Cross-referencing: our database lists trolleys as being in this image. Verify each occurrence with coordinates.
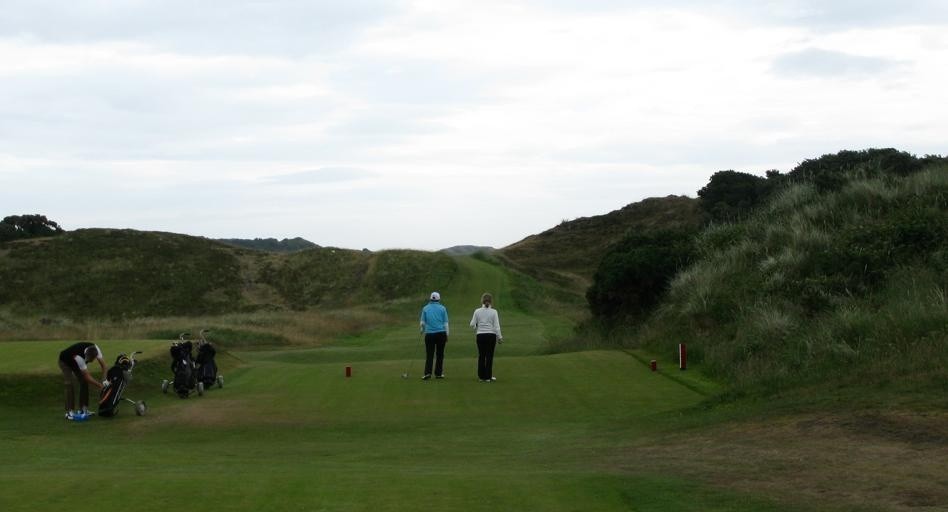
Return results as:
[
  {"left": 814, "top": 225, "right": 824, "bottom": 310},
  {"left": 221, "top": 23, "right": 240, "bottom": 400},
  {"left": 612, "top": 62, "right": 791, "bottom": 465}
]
[
  {"left": 160, "top": 332, "right": 204, "bottom": 400},
  {"left": 97, "top": 348, "right": 146, "bottom": 419},
  {"left": 193, "top": 326, "right": 224, "bottom": 390}
]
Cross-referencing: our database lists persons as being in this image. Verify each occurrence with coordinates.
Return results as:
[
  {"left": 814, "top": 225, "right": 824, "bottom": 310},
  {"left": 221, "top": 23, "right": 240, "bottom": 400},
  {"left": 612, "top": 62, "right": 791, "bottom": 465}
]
[
  {"left": 469, "top": 292, "right": 503, "bottom": 383},
  {"left": 419, "top": 291, "right": 449, "bottom": 380},
  {"left": 58, "top": 341, "right": 109, "bottom": 420}
]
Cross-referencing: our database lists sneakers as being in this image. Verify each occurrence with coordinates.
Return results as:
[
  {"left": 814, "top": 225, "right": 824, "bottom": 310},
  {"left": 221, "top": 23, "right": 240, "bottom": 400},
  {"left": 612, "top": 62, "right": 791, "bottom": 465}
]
[
  {"left": 477, "top": 377, "right": 496, "bottom": 382},
  {"left": 420, "top": 374, "right": 446, "bottom": 380},
  {"left": 64, "top": 408, "right": 96, "bottom": 420}
]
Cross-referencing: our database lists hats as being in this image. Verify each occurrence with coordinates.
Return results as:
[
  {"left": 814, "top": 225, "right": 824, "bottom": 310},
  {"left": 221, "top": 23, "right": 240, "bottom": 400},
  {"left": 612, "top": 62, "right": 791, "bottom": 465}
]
[{"left": 429, "top": 292, "right": 442, "bottom": 301}]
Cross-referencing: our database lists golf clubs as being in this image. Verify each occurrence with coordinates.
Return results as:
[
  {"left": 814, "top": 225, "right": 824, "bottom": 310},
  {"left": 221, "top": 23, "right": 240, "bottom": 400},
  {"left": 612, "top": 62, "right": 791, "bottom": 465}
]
[
  {"left": 401, "top": 334, "right": 421, "bottom": 378},
  {"left": 118, "top": 352, "right": 129, "bottom": 364}
]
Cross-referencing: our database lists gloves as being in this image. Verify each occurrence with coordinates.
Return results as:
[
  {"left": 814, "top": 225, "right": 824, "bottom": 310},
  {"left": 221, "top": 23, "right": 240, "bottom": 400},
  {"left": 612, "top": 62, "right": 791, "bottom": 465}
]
[{"left": 498, "top": 337, "right": 505, "bottom": 344}]
